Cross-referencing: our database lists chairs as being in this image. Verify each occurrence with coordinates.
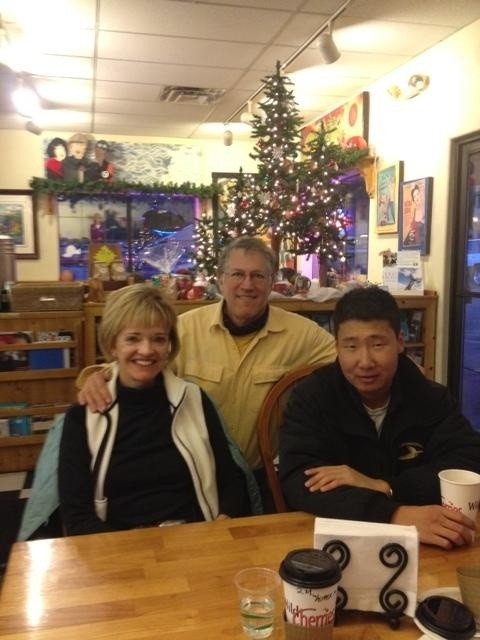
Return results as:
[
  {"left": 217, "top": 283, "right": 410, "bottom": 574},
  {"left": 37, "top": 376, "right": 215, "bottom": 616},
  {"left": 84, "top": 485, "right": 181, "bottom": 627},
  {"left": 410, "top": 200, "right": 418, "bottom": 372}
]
[{"left": 257, "top": 364, "right": 324, "bottom": 511}]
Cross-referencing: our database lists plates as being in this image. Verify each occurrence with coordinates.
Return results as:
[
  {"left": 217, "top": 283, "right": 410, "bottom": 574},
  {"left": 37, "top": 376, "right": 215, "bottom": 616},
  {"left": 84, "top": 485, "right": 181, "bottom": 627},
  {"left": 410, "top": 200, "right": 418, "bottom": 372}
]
[{"left": 415, "top": 587, "right": 480, "bottom": 639}]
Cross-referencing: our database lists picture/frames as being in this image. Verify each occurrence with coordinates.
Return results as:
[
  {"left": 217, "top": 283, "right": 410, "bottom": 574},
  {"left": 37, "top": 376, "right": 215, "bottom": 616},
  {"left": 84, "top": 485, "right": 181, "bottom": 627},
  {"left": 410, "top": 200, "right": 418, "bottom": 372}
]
[
  {"left": 374, "top": 161, "right": 403, "bottom": 235},
  {"left": 0, "top": 189, "right": 39, "bottom": 260},
  {"left": 397, "top": 177, "right": 433, "bottom": 256},
  {"left": 212, "top": 172, "right": 297, "bottom": 276}
]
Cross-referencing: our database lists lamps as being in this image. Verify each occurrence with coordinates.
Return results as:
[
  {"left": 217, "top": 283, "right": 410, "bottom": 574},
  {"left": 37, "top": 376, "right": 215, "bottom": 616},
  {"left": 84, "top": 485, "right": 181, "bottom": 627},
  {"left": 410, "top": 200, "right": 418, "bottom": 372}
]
[
  {"left": 223, "top": 131, "right": 232, "bottom": 144},
  {"left": 316, "top": 21, "right": 340, "bottom": 64},
  {"left": 26, "top": 122, "right": 42, "bottom": 135},
  {"left": 240, "top": 101, "right": 259, "bottom": 127}
]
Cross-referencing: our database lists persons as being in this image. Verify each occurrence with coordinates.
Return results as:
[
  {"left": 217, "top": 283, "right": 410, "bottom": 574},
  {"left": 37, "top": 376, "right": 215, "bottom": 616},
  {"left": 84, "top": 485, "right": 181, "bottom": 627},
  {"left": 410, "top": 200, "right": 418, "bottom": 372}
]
[
  {"left": 74, "top": 232, "right": 339, "bottom": 514},
  {"left": 277, "top": 287, "right": 480, "bottom": 551},
  {"left": 93, "top": 139, "right": 113, "bottom": 186},
  {"left": 404, "top": 184, "right": 425, "bottom": 245},
  {"left": 57, "top": 284, "right": 251, "bottom": 536},
  {"left": 46, "top": 137, "right": 66, "bottom": 180},
  {"left": 63, "top": 133, "right": 110, "bottom": 186}
]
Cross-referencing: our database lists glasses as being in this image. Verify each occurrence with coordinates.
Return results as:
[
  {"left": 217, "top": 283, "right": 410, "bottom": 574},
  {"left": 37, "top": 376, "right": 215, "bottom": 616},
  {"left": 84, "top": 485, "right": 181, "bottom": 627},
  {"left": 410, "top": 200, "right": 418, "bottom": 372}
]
[{"left": 222, "top": 269, "right": 275, "bottom": 284}]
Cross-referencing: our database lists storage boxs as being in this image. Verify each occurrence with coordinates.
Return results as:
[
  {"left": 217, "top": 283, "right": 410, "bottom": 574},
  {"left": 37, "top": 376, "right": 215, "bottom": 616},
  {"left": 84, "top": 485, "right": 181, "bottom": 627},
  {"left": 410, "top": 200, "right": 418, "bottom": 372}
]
[{"left": 10, "top": 281, "right": 83, "bottom": 311}]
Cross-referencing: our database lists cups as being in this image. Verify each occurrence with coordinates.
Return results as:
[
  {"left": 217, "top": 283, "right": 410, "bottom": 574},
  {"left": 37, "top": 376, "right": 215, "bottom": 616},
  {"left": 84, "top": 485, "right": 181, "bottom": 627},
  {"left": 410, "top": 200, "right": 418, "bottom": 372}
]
[
  {"left": 456, "top": 565, "right": 480, "bottom": 626},
  {"left": 232, "top": 566, "right": 281, "bottom": 637},
  {"left": 278, "top": 549, "right": 342, "bottom": 639},
  {"left": 437, "top": 469, "right": 480, "bottom": 526}
]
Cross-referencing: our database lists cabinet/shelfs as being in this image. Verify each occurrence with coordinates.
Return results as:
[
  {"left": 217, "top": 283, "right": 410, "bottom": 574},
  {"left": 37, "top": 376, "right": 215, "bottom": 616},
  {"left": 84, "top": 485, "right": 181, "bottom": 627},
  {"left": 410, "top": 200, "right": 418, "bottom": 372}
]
[
  {"left": 0, "top": 310, "right": 86, "bottom": 474},
  {"left": 87, "top": 290, "right": 438, "bottom": 381}
]
